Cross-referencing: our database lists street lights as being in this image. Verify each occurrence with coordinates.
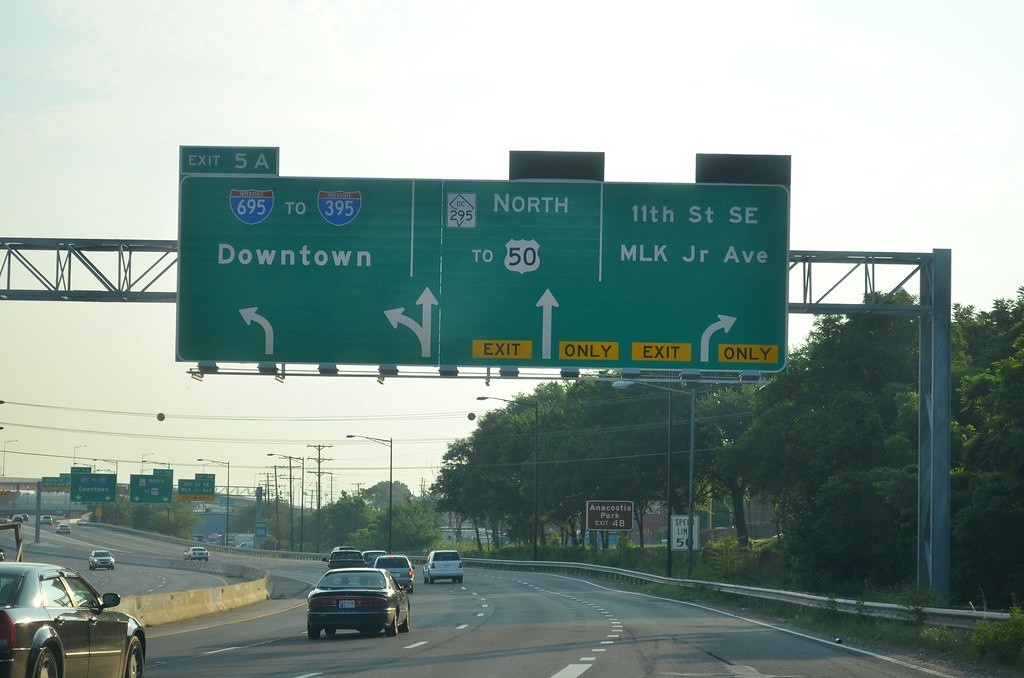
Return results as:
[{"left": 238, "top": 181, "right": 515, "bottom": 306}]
[
  {"left": 196, "top": 458, "right": 230, "bottom": 547},
  {"left": 345, "top": 434, "right": 393, "bottom": 554},
  {"left": 611, "top": 380, "right": 697, "bottom": 581},
  {"left": 2, "top": 439, "right": 19, "bottom": 478},
  {"left": 141, "top": 460, "right": 170, "bottom": 537},
  {"left": 92, "top": 458, "right": 118, "bottom": 476},
  {"left": 73, "top": 444, "right": 88, "bottom": 464},
  {"left": 475, "top": 396, "right": 539, "bottom": 562},
  {"left": 74, "top": 462, "right": 95, "bottom": 473},
  {"left": 267, "top": 453, "right": 304, "bottom": 553}
]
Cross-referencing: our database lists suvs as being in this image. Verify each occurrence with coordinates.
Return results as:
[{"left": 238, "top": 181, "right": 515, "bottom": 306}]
[
  {"left": 361, "top": 550, "right": 388, "bottom": 568},
  {"left": 423, "top": 549, "right": 463, "bottom": 584},
  {"left": 333, "top": 545, "right": 356, "bottom": 550},
  {"left": 89, "top": 549, "right": 115, "bottom": 571},
  {"left": 321, "top": 550, "right": 367, "bottom": 570},
  {"left": 374, "top": 555, "right": 415, "bottom": 593}
]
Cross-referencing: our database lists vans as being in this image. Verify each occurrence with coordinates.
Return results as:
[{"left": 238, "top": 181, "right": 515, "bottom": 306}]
[{"left": 236, "top": 542, "right": 253, "bottom": 548}]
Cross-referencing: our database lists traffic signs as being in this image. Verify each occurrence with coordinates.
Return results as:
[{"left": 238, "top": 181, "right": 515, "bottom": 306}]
[
  {"left": 443, "top": 180, "right": 601, "bottom": 367},
  {"left": 129, "top": 474, "right": 172, "bottom": 505},
  {"left": 70, "top": 473, "right": 117, "bottom": 503},
  {"left": 174, "top": 174, "right": 443, "bottom": 368},
  {"left": 600, "top": 180, "right": 790, "bottom": 373}
]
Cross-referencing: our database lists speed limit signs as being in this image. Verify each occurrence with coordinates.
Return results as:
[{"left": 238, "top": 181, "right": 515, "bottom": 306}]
[{"left": 670, "top": 514, "right": 701, "bottom": 552}]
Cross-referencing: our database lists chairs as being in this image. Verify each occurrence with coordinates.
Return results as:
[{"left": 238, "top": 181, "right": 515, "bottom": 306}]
[
  {"left": 399, "top": 560, "right": 406, "bottom": 568},
  {"left": 341, "top": 577, "right": 350, "bottom": 586},
  {"left": 359, "top": 576, "right": 369, "bottom": 586},
  {"left": 199, "top": 549, "right": 203, "bottom": 551},
  {"left": 451, "top": 555, "right": 458, "bottom": 560}
]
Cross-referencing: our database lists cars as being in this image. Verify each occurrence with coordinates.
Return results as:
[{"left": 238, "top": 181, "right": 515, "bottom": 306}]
[
  {"left": 307, "top": 567, "right": 411, "bottom": 639},
  {"left": 55, "top": 523, "right": 71, "bottom": 534},
  {"left": 39, "top": 515, "right": 53, "bottom": 526},
  {"left": 11, "top": 514, "right": 24, "bottom": 523},
  {"left": 183, "top": 547, "right": 209, "bottom": 562},
  {"left": 22, "top": 514, "right": 29, "bottom": 522},
  {"left": 0, "top": 561, "right": 147, "bottom": 678}
]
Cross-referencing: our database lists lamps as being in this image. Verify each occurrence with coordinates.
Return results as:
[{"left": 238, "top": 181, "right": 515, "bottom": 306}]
[
  {"left": 679, "top": 369, "right": 703, "bottom": 381},
  {"left": 378, "top": 364, "right": 399, "bottom": 375},
  {"left": 197, "top": 361, "right": 219, "bottom": 373},
  {"left": 257, "top": 362, "right": 279, "bottom": 374},
  {"left": 499, "top": 366, "right": 519, "bottom": 378},
  {"left": 317, "top": 364, "right": 339, "bottom": 375},
  {"left": 438, "top": 366, "right": 459, "bottom": 377},
  {"left": 560, "top": 368, "right": 580, "bottom": 377},
  {"left": 739, "top": 371, "right": 760, "bottom": 382},
  {"left": 621, "top": 367, "right": 641, "bottom": 378}
]
